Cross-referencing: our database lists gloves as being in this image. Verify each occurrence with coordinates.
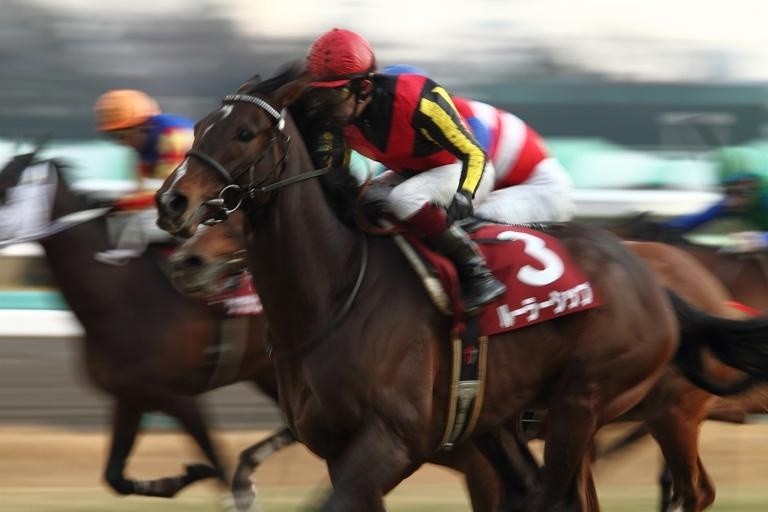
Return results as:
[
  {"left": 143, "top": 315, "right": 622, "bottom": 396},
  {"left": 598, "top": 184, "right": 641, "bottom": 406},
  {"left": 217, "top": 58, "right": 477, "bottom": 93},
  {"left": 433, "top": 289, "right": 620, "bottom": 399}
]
[{"left": 445, "top": 188, "right": 475, "bottom": 228}]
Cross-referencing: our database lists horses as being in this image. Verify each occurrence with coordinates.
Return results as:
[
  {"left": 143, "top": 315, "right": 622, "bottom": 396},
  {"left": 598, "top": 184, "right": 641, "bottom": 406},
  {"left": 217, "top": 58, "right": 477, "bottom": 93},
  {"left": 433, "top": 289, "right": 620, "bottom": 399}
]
[
  {"left": 0, "top": 148, "right": 278, "bottom": 500},
  {"left": 154, "top": 72, "right": 767, "bottom": 512},
  {"left": 548, "top": 214, "right": 767, "bottom": 512}
]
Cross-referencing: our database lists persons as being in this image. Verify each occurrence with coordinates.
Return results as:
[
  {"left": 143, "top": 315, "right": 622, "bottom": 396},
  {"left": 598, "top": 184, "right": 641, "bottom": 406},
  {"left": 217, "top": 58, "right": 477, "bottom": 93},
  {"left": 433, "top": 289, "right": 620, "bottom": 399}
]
[
  {"left": 302, "top": 26, "right": 575, "bottom": 326},
  {"left": 94, "top": 88, "right": 265, "bottom": 318},
  {"left": 640, "top": 141, "right": 766, "bottom": 256}
]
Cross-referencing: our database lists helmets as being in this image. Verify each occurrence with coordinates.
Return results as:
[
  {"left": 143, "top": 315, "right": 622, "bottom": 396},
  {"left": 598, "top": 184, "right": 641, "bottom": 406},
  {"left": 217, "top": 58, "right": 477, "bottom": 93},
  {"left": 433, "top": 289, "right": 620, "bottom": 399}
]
[
  {"left": 297, "top": 26, "right": 378, "bottom": 88},
  {"left": 92, "top": 89, "right": 161, "bottom": 133}
]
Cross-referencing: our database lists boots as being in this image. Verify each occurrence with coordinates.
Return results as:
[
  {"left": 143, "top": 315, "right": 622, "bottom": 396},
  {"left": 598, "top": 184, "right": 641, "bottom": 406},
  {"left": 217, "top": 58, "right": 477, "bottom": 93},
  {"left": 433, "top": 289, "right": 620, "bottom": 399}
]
[{"left": 428, "top": 221, "right": 508, "bottom": 314}]
